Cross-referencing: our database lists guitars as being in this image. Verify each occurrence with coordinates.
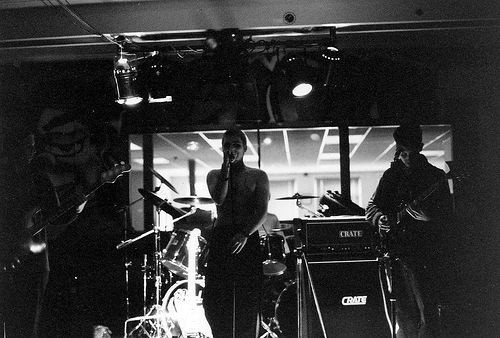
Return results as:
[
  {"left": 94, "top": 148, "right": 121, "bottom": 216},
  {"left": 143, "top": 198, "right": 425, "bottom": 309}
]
[
  {"left": 382, "top": 165, "right": 466, "bottom": 241},
  {"left": 0, "top": 160, "right": 132, "bottom": 271}
]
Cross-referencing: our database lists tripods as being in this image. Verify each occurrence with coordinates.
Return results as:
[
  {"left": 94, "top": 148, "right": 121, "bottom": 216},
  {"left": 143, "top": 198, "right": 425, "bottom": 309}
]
[{"left": 124, "top": 204, "right": 183, "bottom": 338}]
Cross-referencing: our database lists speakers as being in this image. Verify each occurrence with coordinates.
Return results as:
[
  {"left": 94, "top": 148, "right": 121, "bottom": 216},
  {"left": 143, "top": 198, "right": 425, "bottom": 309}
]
[{"left": 304, "top": 254, "right": 396, "bottom": 337}]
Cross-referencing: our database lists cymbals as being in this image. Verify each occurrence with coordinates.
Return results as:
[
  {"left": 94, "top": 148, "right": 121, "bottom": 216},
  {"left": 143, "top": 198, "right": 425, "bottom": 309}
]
[
  {"left": 274, "top": 194, "right": 321, "bottom": 201},
  {"left": 173, "top": 196, "right": 214, "bottom": 206},
  {"left": 144, "top": 163, "right": 179, "bottom": 194},
  {"left": 137, "top": 188, "right": 186, "bottom": 218}
]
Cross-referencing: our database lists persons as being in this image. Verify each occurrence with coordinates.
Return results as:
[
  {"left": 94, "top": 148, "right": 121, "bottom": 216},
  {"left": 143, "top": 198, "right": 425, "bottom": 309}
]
[
  {"left": 365, "top": 128, "right": 454, "bottom": 338},
  {"left": 206, "top": 126, "right": 290, "bottom": 338},
  {"left": 0, "top": 129, "right": 89, "bottom": 338}
]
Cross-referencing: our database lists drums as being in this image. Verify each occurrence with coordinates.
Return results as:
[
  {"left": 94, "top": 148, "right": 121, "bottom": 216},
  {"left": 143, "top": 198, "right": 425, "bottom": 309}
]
[
  {"left": 161, "top": 279, "right": 214, "bottom": 338},
  {"left": 259, "top": 232, "right": 288, "bottom": 277},
  {"left": 156, "top": 228, "right": 210, "bottom": 279}
]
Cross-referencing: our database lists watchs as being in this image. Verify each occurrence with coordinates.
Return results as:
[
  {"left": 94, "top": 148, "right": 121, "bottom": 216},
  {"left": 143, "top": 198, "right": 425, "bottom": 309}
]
[{"left": 219, "top": 173, "right": 230, "bottom": 182}]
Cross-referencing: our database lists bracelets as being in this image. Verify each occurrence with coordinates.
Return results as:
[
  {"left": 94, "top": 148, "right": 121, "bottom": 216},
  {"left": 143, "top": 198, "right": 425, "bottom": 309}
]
[{"left": 241, "top": 228, "right": 250, "bottom": 237}]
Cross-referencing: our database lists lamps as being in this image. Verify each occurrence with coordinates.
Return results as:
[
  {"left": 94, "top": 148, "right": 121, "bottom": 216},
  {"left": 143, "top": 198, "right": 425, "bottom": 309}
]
[
  {"left": 202, "top": 33, "right": 224, "bottom": 54},
  {"left": 113, "top": 50, "right": 144, "bottom": 106},
  {"left": 143, "top": 51, "right": 173, "bottom": 103},
  {"left": 319, "top": 28, "right": 346, "bottom": 63},
  {"left": 276, "top": 45, "right": 313, "bottom": 97}
]
